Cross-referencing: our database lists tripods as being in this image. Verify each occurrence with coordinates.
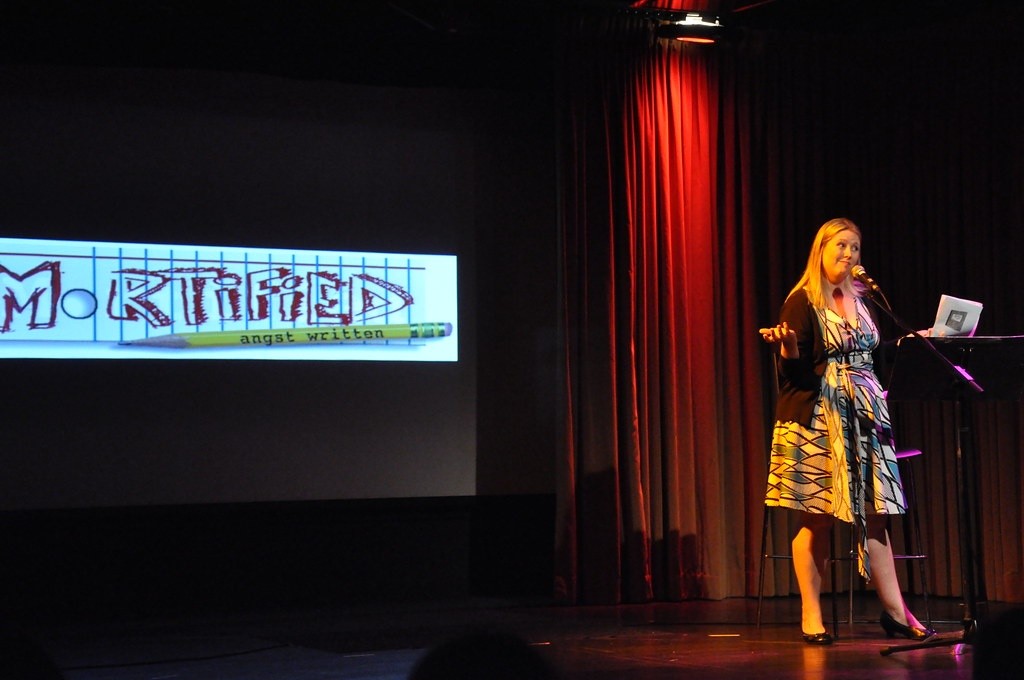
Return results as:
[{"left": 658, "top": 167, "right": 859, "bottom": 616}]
[{"left": 863, "top": 287, "right": 986, "bottom": 656}]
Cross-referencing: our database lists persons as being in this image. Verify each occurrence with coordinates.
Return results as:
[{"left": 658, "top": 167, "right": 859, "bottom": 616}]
[
  {"left": 408, "top": 632, "right": 557, "bottom": 680},
  {"left": 759, "top": 217, "right": 944, "bottom": 644},
  {"left": 0, "top": 612, "right": 62, "bottom": 680},
  {"left": 972, "top": 608, "right": 1024, "bottom": 679}
]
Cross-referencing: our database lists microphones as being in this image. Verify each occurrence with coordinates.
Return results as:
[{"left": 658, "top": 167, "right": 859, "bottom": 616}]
[{"left": 852, "top": 265, "right": 881, "bottom": 292}]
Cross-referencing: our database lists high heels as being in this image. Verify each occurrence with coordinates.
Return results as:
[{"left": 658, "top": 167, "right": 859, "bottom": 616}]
[
  {"left": 802, "top": 621, "right": 832, "bottom": 646},
  {"left": 880, "top": 610, "right": 938, "bottom": 640}
]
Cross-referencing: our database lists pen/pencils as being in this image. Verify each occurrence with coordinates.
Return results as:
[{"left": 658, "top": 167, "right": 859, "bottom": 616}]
[{"left": 116, "top": 320, "right": 455, "bottom": 350}]
